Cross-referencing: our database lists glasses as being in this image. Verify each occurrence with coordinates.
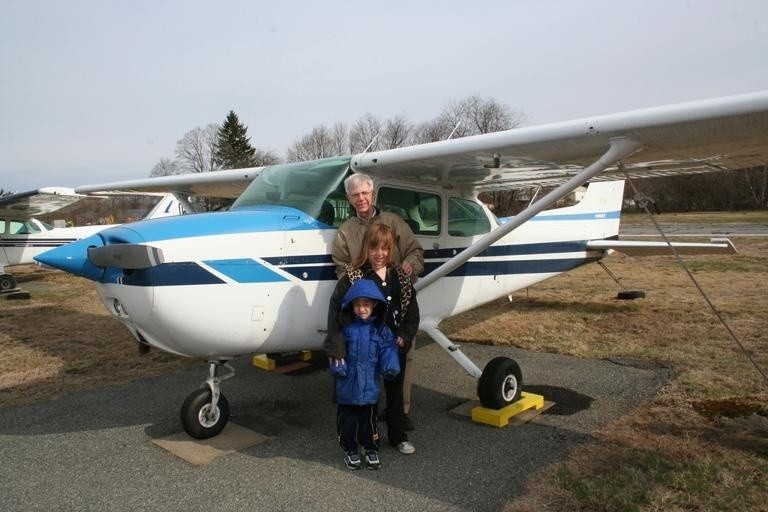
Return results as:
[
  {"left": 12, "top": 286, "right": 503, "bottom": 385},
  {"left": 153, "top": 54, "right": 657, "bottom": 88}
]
[{"left": 347, "top": 191, "right": 371, "bottom": 197}]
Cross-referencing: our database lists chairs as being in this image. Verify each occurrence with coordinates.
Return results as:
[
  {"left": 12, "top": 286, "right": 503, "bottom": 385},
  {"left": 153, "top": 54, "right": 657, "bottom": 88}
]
[{"left": 315, "top": 201, "right": 335, "bottom": 225}]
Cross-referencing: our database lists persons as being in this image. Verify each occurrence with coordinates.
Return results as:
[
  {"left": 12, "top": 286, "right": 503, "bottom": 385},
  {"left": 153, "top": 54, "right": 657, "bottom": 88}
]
[{"left": 323, "top": 173, "right": 424, "bottom": 470}]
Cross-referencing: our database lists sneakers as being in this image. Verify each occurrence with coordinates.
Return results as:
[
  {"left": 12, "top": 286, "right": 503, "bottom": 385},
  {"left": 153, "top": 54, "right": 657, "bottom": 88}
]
[
  {"left": 401, "top": 416, "right": 415, "bottom": 432},
  {"left": 391, "top": 437, "right": 416, "bottom": 455},
  {"left": 343, "top": 444, "right": 382, "bottom": 470}
]
